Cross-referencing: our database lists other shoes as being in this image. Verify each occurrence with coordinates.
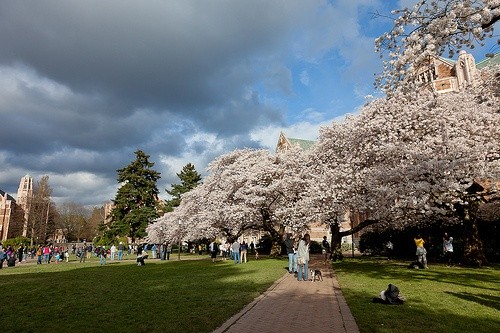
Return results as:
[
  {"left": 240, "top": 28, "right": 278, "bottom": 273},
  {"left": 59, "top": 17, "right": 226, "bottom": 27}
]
[
  {"left": 289, "top": 271, "right": 293, "bottom": 274},
  {"left": 294, "top": 271, "right": 298, "bottom": 273}
]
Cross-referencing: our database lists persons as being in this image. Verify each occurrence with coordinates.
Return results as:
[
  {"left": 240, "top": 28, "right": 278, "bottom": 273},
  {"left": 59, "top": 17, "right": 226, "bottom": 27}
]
[
  {"left": 0, "top": 241, "right": 149, "bottom": 270},
  {"left": 373, "top": 284, "right": 407, "bottom": 306},
  {"left": 206, "top": 240, "right": 260, "bottom": 264},
  {"left": 190, "top": 243, "right": 194, "bottom": 255},
  {"left": 199, "top": 244, "right": 204, "bottom": 256},
  {"left": 442, "top": 232, "right": 456, "bottom": 267},
  {"left": 285, "top": 232, "right": 311, "bottom": 281},
  {"left": 152, "top": 241, "right": 172, "bottom": 260},
  {"left": 413, "top": 233, "right": 429, "bottom": 269},
  {"left": 386, "top": 240, "right": 394, "bottom": 261},
  {"left": 360, "top": 239, "right": 367, "bottom": 255},
  {"left": 321, "top": 236, "right": 331, "bottom": 262}
]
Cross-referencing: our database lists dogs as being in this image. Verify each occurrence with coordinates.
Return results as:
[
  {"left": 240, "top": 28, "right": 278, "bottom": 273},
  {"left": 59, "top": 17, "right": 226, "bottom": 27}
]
[{"left": 310, "top": 268, "right": 323, "bottom": 283}]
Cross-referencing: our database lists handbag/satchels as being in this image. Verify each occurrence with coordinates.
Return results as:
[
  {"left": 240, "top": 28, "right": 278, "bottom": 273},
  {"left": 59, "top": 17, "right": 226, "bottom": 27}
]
[{"left": 297, "top": 256, "right": 306, "bottom": 265}]
